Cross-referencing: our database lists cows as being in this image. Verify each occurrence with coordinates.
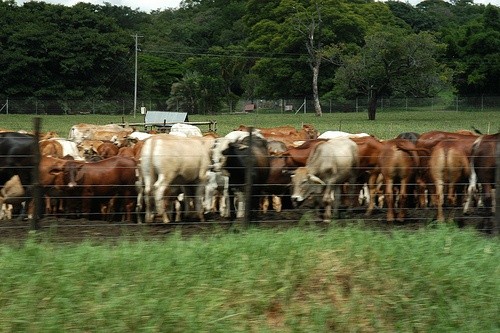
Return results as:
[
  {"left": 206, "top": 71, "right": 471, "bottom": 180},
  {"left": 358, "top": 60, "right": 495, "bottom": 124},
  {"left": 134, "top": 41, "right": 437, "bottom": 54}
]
[{"left": 0, "top": 123, "right": 500, "bottom": 234}]
